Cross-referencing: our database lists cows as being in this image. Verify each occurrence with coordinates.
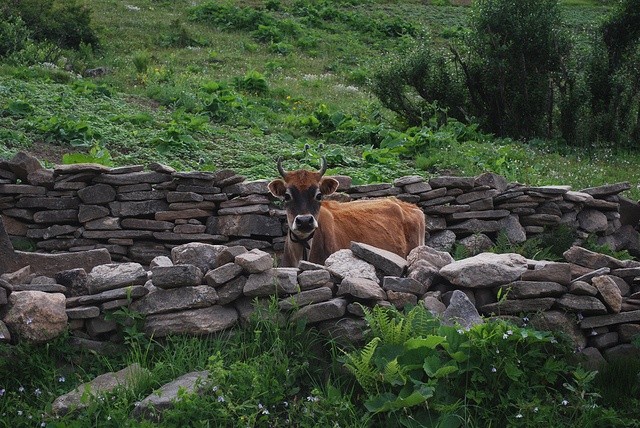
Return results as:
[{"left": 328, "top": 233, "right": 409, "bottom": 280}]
[{"left": 267, "top": 155, "right": 426, "bottom": 267}]
